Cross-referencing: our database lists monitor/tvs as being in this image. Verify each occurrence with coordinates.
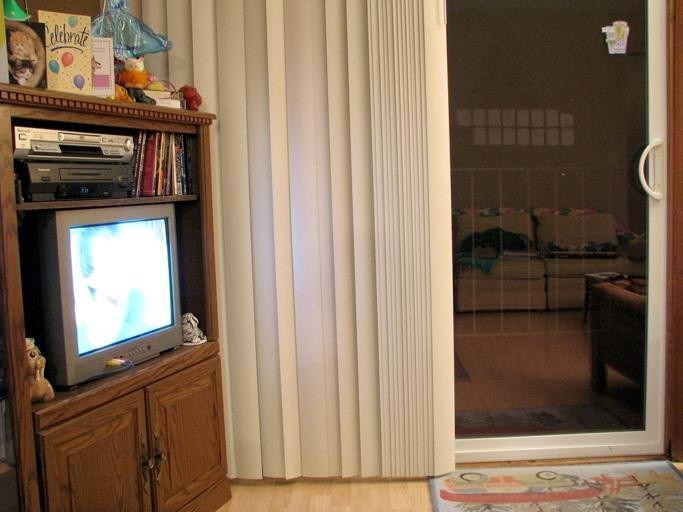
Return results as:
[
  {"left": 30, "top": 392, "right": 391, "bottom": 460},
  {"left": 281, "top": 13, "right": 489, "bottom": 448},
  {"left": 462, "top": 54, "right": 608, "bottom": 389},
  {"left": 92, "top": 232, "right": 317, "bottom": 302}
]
[{"left": 41, "top": 204, "right": 185, "bottom": 388}]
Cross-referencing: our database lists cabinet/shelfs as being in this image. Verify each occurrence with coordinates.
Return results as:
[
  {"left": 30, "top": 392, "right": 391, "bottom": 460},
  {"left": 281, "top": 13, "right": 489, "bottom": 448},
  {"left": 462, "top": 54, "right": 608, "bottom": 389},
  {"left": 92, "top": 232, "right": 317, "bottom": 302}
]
[{"left": 0, "top": 83, "right": 233, "bottom": 512}]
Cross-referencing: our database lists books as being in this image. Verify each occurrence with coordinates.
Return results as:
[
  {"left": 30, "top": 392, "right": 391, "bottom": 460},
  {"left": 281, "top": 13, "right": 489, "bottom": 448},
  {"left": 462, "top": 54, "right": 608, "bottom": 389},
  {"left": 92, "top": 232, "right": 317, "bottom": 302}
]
[
  {"left": 131, "top": 132, "right": 195, "bottom": 196},
  {"left": 138, "top": 98, "right": 186, "bottom": 109},
  {"left": 130, "top": 89, "right": 183, "bottom": 100}
]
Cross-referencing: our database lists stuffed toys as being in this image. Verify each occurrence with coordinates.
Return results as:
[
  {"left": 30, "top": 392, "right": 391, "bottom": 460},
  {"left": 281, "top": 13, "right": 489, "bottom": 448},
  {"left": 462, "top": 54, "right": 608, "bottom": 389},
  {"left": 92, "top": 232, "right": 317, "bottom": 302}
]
[{"left": 115, "top": 58, "right": 154, "bottom": 88}]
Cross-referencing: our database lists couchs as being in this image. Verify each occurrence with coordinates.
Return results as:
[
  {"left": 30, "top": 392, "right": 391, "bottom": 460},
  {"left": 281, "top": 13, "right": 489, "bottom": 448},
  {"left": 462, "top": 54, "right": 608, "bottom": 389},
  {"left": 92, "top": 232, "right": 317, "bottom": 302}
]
[{"left": 446, "top": 208, "right": 646, "bottom": 403}]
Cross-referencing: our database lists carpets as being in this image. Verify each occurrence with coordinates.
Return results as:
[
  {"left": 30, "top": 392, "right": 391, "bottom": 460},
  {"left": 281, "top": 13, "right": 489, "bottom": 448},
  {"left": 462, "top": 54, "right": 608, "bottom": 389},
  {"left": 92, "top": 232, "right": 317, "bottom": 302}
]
[{"left": 428, "top": 458, "right": 683, "bottom": 512}]
